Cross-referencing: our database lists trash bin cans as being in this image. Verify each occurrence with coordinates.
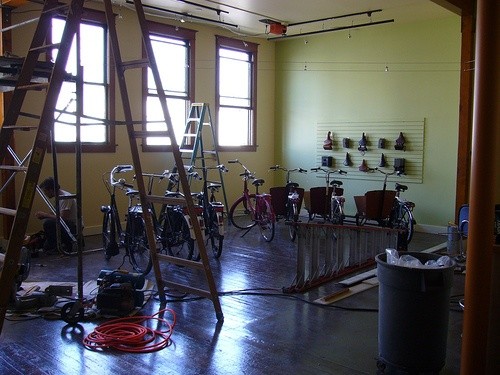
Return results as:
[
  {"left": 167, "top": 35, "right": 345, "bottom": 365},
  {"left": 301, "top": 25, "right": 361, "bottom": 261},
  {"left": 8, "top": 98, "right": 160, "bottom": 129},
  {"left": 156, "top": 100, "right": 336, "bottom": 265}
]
[{"left": 375, "top": 251, "right": 456, "bottom": 371}]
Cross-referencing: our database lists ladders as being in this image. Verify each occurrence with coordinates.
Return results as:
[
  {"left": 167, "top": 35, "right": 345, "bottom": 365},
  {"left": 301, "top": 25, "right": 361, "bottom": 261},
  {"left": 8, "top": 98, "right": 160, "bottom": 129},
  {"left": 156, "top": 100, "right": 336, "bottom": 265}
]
[
  {"left": 156, "top": 103, "right": 232, "bottom": 227},
  {"left": 0, "top": 0, "right": 224, "bottom": 323}
]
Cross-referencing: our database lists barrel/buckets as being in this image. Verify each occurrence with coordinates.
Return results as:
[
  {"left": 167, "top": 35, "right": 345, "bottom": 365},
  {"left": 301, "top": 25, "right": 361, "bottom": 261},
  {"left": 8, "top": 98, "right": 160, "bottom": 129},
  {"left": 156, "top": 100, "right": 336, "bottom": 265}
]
[{"left": 375, "top": 251, "right": 456, "bottom": 374}]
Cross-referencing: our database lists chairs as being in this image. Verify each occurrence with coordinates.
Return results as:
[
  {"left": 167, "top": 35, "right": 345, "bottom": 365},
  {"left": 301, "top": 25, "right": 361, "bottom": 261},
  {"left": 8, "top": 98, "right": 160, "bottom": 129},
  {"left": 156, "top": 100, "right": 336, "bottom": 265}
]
[{"left": 457, "top": 204, "right": 469, "bottom": 258}]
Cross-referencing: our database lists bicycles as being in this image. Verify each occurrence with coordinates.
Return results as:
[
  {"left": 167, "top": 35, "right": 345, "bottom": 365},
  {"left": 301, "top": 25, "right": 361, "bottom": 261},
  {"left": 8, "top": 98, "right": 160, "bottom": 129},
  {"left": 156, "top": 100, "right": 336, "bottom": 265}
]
[
  {"left": 227, "top": 159, "right": 276, "bottom": 243},
  {"left": 100, "top": 165, "right": 153, "bottom": 277},
  {"left": 368, "top": 167, "right": 417, "bottom": 248},
  {"left": 269, "top": 165, "right": 308, "bottom": 241},
  {"left": 310, "top": 167, "right": 348, "bottom": 240},
  {"left": 186, "top": 165, "right": 230, "bottom": 259},
  {"left": 131, "top": 172, "right": 197, "bottom": 268}
]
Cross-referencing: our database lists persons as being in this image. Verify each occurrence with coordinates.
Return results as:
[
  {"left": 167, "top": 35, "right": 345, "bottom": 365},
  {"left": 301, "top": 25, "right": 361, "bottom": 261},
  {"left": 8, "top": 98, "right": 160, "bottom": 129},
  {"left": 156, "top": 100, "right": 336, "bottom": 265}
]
[{"left": 36, "top": 177, "right": 84, "bottom": 259}]
